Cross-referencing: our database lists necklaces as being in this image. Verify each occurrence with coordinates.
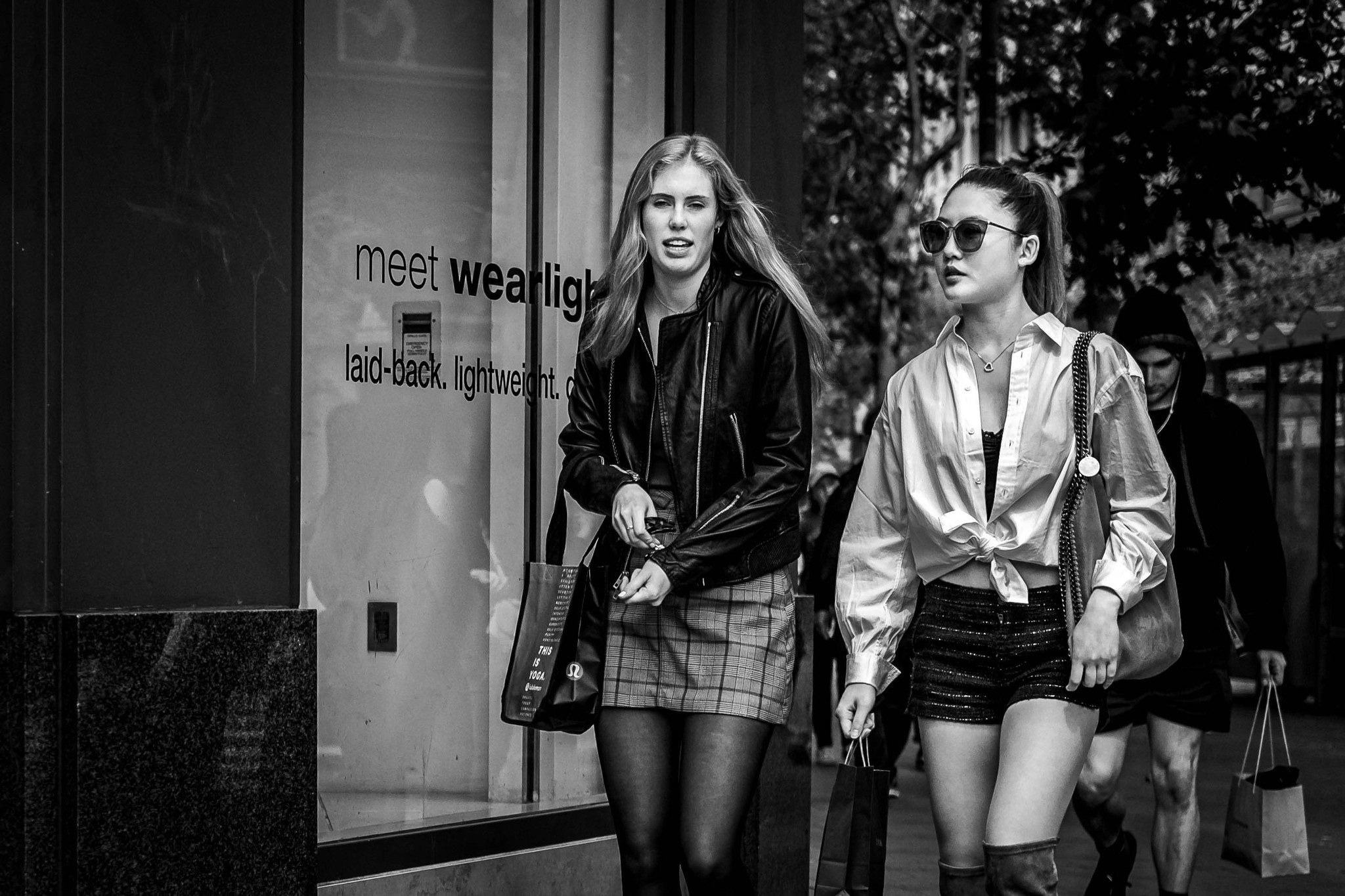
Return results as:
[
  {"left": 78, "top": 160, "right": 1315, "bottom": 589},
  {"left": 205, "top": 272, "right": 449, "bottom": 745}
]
[
  {"left": 968, "top": 339, "right": 1017, "bottom": 371},
  {"left": 652, "top": 286, "right": 696, "bottom": 314}
]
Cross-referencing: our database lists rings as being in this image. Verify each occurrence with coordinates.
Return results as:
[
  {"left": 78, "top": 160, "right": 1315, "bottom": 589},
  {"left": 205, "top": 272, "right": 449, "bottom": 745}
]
[{"left": 626, "top": 525, "right": 633, "bottom": 530}]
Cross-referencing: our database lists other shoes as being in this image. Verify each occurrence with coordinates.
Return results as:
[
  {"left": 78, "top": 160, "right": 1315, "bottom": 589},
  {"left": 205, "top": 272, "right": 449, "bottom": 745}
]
[
  {"left": 1084, "top": 830, "right": 1137, "bottom": 896},
  {"left": 937, "top": 859, "right": 986, "bottom": 896},
  {"left": 982, "top": 837, "right": 1060, "bottom": 896}
]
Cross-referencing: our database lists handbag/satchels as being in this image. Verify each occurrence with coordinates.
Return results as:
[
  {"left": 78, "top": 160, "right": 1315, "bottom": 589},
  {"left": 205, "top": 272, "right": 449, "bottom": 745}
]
[
  {"left": 1057, "top": 328, "right": 1184, "bottom": 679},
  {"left": 501, "top": 450, "right": 613, "bottom": 735},
  {"left": 1221, "top": 677, "right": 1310, "bottom": 878},
  {"left": 813, "top": 735, "right": 890, "bottom": 896}
]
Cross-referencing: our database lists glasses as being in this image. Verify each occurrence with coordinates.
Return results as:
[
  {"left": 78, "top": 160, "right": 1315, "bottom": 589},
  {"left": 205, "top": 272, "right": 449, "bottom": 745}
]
[
  {"left": 919, "top": 218, "right": 1029, "bottom": 254},
  {"left": 615, "top": 517, "right": 677, "bottom": 546}
]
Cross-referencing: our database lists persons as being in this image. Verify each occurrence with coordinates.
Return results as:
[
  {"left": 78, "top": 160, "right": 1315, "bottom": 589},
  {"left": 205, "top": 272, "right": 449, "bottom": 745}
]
[
  {"left": 801, "top": 403, "right": 926, "bottom": 797},
  {"left": 1073, "top": 285, "right": 1291, "bottom": 896},
  {"left": 556, "top": 134, "right": 834, "bottom": 896},
  {"left": 835, "top": 165, "right": 1178, "bottom": 896}
]
